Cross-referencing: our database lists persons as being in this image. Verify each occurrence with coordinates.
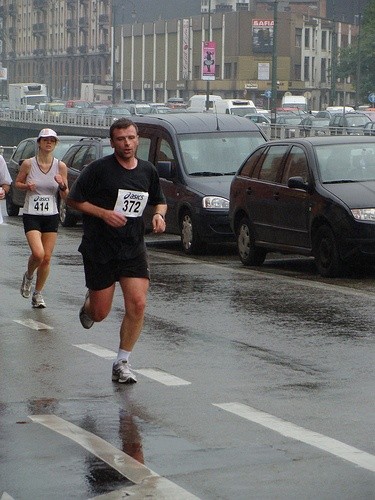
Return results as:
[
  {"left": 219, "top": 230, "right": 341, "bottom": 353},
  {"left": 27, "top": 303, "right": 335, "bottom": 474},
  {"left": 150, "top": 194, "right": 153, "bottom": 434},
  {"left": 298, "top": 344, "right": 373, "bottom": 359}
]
[
  {"left": 0, "top": 154, "right": 13, "bottom": 224},
  {"left": 15, "top": 128, "right": 70, "bottom": 309},
  {"left": 66, "top": 117, "right": 168, "bottom": 384}
]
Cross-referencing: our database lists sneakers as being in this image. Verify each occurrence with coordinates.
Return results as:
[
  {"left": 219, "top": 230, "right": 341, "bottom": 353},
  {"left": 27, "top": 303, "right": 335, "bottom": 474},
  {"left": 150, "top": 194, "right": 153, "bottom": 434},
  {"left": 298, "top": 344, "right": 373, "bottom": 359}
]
[
  {"left": 112, "top": 358, "right": 137, "bottom": 383},
  {"left": 20, "top": 271, "right": 33, "bottom": 298},
  {"left": 79, "top": 290, "right": 94, "bottom": 329},
  {"left": 32, "top": 292, "right": 46, "bottom": 308}
]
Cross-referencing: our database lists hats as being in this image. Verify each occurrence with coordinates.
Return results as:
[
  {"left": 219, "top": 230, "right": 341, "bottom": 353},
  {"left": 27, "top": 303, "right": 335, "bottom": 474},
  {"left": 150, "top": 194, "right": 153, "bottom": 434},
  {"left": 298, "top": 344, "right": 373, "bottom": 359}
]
[{"left": 37, "top": 129, "right": 58, "bottom": 142}]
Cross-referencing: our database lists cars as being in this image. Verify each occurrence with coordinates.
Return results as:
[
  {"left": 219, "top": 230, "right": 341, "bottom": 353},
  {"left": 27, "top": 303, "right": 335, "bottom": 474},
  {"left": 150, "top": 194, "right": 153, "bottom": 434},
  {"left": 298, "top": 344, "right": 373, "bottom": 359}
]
[
  {"left": 1, "top": 83, "right": 375, "bottom": 139},
  {"left": 115, "top": 112, "right": 270, "bottom": 256},
  {"left": 228, "top": 135, "right": 375, "bottom": 279},
  {"left": 5, "top": 135, "right": 114, "bottom": 225}
]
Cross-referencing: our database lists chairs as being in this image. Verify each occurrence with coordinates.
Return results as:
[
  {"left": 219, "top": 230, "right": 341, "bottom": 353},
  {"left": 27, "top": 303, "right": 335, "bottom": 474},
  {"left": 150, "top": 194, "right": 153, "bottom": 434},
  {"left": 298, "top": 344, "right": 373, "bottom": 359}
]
[{"left": 327, "top": 152, "right": 351, "bottom": 178}]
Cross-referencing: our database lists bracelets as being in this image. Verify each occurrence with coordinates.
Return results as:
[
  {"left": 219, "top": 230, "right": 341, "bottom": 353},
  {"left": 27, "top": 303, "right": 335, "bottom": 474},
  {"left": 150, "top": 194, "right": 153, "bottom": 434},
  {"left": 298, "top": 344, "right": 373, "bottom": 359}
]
[
  {"left": 59, "top": 183, "right": 67, "bottom": 192},
  {"left": 154, "top": 212, "right": 164, "bottom": 219}
]
